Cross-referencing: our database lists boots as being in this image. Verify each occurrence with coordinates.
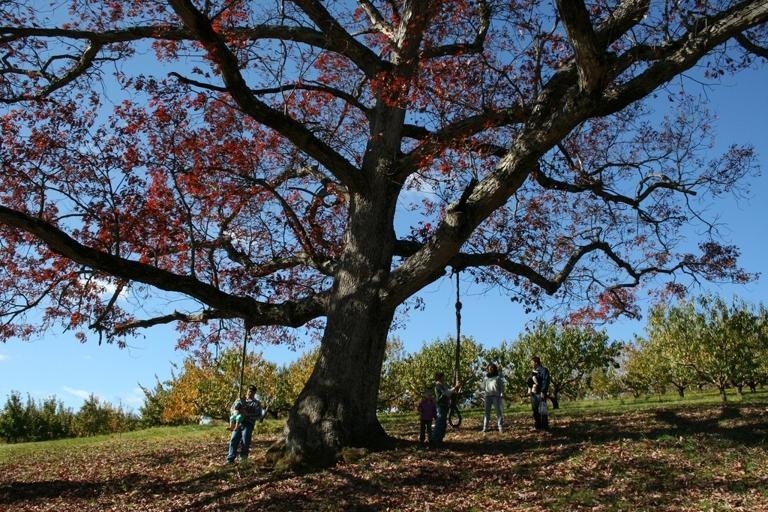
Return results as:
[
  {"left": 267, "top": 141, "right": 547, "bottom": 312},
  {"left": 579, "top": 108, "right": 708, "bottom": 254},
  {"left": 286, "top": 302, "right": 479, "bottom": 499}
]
[
  {"left": 498, "top": 416, "right": 504, "bottom": 432},
  {"left": 482, "top": 415, "right": 488, "bottom": 432}
]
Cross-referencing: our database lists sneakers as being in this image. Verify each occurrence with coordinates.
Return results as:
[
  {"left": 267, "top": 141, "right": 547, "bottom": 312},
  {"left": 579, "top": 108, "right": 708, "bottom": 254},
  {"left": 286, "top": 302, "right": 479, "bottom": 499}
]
[{"left": 225, "top": 426, "right": 239, "bottom": 431}]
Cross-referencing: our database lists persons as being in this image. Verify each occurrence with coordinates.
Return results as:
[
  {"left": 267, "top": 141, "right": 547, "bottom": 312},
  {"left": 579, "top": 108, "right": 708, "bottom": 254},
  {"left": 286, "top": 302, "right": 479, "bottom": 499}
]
[
  {"left": 476, "top": 362, "right": 507, "bottom": 432},
  {"left": 226, "top": 390, "right": 249, "bottom": 431},
  {"left": 433, "top": 371, "right": 461, "bottom": 442},
  {"left": 224, "top": 383, "right": 259, "bottom": 466},
  {"left": 419, "top": 391, "right": 439, "bottom": 442},
  {"left": 525, "top": 356, "right": 550, "bottom": 431}
]
